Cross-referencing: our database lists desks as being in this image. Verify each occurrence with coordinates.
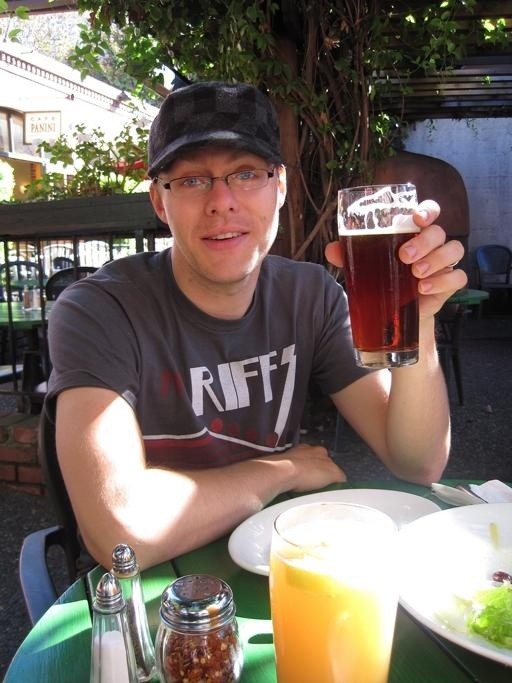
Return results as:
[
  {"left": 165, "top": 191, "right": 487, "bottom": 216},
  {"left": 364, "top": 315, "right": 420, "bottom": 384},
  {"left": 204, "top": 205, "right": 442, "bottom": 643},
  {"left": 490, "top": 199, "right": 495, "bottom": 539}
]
[{"left": 435, "top": 288, "right": 491, "bottom": 406}]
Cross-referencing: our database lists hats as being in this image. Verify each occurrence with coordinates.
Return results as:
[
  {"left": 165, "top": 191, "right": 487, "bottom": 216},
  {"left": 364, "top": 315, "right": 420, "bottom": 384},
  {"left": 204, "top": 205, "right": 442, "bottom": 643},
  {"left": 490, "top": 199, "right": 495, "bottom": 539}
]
[{"left": 147, "top": 80, "right": 285, "bottom": 178}]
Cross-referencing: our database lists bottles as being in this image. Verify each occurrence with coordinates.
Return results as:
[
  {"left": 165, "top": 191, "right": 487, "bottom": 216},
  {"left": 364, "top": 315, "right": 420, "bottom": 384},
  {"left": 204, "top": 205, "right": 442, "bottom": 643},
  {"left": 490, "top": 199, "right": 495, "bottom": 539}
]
[
  {"left": 269, "top": 502, "right": 403, "bottom": 683},
  {"left": 89, "top": 543, "right": 243, "bottom": 683},
  {"left": 22, "top": 285, "right": 40, "bottom": 309}
]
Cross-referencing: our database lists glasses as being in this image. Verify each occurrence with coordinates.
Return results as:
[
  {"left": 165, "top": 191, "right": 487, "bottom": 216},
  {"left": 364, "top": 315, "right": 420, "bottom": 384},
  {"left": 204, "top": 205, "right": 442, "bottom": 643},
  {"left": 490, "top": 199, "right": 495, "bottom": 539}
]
[{"left": 153, "top": 164, "right": 277, "bottom": 198}]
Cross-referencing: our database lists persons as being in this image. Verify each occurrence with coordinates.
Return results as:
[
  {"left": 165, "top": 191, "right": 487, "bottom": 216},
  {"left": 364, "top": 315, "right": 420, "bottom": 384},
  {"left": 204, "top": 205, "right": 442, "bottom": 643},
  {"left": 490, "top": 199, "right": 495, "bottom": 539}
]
[{"left": 42, "top": 78, "right": 469, "bottom": 581}]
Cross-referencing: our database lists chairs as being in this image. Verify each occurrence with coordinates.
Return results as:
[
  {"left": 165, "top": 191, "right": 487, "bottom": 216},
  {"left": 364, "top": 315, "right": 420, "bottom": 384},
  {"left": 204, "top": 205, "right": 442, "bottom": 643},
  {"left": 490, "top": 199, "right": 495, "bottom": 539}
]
[
  {"left": 0, "top": 258, "right": 100, "bottom": 416},
  {"left": 477, "top": 244, "right": 512, "bottom": 292},
  {"left": 18, "top": 406, "right": 97, "bottom": 627}
]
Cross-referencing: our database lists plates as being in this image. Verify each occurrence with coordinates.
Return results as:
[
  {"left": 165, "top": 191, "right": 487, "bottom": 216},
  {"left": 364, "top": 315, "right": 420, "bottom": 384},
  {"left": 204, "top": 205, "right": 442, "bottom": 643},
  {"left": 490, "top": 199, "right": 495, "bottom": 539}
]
[
  {"left": 398, "top": 502, "right": 512, "bottom": 665},
  {"left": 228, "top": 491, "right": 440, "bottom": 578}
]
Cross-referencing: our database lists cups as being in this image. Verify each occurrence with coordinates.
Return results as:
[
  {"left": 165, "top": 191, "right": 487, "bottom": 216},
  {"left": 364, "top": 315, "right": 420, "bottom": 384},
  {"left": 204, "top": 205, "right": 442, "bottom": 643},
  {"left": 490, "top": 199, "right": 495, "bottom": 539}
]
[{"left": 338, "top": 183, "right": 421, "bottom": 368}]
